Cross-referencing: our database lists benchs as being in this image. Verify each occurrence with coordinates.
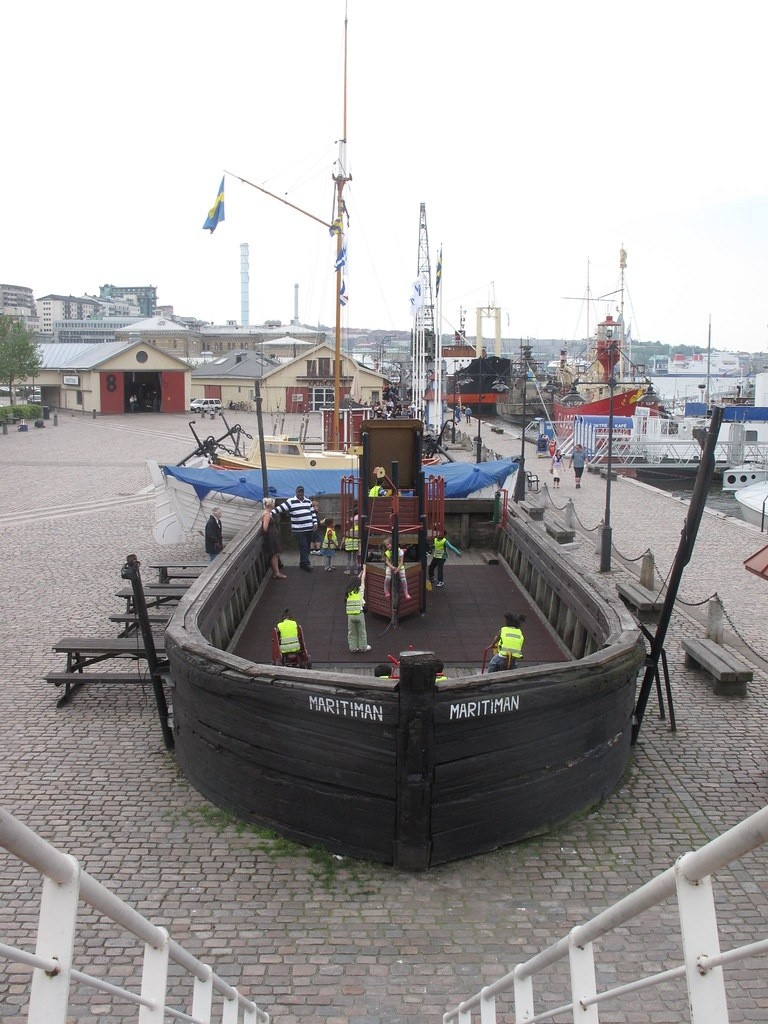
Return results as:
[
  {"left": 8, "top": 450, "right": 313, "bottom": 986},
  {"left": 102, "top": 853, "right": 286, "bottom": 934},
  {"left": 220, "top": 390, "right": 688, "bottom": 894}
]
[
  {"left": 599, "top": 469, "right": 618, "bottom": 482},
  {"left": 616, "top": 582, "right": 664, "bottom": 623},
  {"left": 544, "top": 519, "right": 575, "bottom": 544},
  {"left": 681, "top": 639, "right": 753, "bottom": 697},
  {"left": 588, "top": 464, "right": 604, "bottom": 474},
  {"left": 0, "top": 413, "right": 20, "bottom": 426},
  {"left": 490, "top": 426, "right": 504, "bottom": 434},
  {"left": 43, "top": 573, "right": 202, "bottom": 686},
  {"left": 519, "top": 501, "right": 545, "bottom": 521}
]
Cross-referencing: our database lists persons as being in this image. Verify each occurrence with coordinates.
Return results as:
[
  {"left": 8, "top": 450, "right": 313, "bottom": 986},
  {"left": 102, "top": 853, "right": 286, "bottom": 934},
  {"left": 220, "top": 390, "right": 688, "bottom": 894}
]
[
  {"left": 130, "top": 394, "right": 137, "bottom": 412},
  {"left": 226, "top": 401, "right": 233, "bottom": 410},
  {"left": 487, "top": 613, "right": 525, "bottom": 673},
  {"left": 270, "top": 486, "right": 318, "bottom": 572},
  {"left": 205, "top": 508, "right": 223, "bottom": 561},
  {"left": 369, "top": 478, "right": 387, "bottom": 497},
  {"left": 345, "top": 564, "right": 371, "bottom": 652},
  {"left": 276, "top": 608, "right": 301, "bottom": 654},
  {"left": 310, "top": 501, "right": 339, "bottom": 571},
  {"left": 374, "top": 664, "right": 392, "bottom": 678},
  {"left": 550, "top": 448, "right": 566, "bottom": 488},
  {"left": 383, "top": 538, "right": 413, "bottom": 600},
  {"left": 428, "top": 529, "right": 462, "bottom": 587},
  {"left": 262, "top": 498, "right": 287, "bottom": 579},
  {"left": 339, "top": 515, "right": 362, "bottom": 574},
  {"left": 569, "top": 444, "right": 589, "bottom": 490},
  {"left": 455, "top": 404, "right": 460, "bottom": 422},
  {"left": 465, "top": 406, "right": 472, "bottom": 423},
  {"left": 435, "top": 658, "right": 452, "bottom": 684},
  {"left": 371, "top": 386, "right": 412, "bottom": 420}
]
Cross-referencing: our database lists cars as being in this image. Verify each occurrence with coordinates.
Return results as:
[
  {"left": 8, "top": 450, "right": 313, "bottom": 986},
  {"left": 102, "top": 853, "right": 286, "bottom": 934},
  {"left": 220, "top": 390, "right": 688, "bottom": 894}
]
[{"left": 0, "top": 385, "right": 41, "bottom": 404}]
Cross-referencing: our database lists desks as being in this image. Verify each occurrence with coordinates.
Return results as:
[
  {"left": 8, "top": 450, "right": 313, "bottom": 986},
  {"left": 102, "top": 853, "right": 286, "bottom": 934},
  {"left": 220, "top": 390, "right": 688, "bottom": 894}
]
[
  {"left": 52, "top": 636, "right": 169, "bottom": 708},
  {"left": 149, "top": 561, "right": 210, "bottom": 609},
  {"left": 116, "top": 587, "right": 188, "bottom": 638}
]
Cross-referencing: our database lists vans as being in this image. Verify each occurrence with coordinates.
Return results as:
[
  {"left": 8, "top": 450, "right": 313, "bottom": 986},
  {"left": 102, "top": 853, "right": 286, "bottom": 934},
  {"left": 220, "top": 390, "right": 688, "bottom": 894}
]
[{"left": 190, "top": 398, "right": 222, "bottom": 413}]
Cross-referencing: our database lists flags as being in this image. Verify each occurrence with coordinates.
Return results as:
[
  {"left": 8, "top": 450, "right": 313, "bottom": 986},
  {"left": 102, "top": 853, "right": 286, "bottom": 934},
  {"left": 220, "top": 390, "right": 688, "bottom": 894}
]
[
  {"left": 202, "top": 174, "right": 225, "bottom": 234},
  {"left": 435, "top": 243, "right": 442, "bottom": 298}
]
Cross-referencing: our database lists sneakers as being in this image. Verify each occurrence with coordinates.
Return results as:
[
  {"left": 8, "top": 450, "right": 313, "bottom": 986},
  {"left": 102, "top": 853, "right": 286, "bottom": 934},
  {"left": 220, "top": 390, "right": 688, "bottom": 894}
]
[
  {"left": 437, "top": 581, "right": 444, "bottom": 587},
  {"left": 428, "top": 576, "right": 436, "bottom": 582}
]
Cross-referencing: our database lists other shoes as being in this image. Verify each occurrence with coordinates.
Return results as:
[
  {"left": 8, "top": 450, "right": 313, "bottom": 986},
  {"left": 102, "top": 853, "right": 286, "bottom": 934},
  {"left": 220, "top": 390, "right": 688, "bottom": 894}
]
[
  {"left": 273, "top": 574, "right": 287, "bottom": 579},
  {"left": 344, "top": 569, "right": 351, "bottom": 575},
  {"left": 310, "top": 549, "right": 317, "bottom": 555},
  {"left": 318, "top": 550, "right": 322, "bottom": 556},
  {"left": 300, "top": 563, "right": 314, "bottom": 570},
  {"left": 362, "top": 644, "right": 371, "bottom": 652},
  {"left": 405, "top": 593, "right": 412, "bottom": 600},
  {"left": 324, "top": 565, "right": 336, "bottom": 572},
  {"left": 385, "top": 591, "right": 391, "bottom": 600},
  {"left": 354, "top": 570, "right": 358, "bottom": 575}
]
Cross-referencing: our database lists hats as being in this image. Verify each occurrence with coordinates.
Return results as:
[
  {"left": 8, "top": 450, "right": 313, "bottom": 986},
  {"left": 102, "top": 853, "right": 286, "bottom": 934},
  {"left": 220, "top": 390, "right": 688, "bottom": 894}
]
[
  {"left": 297, "top": 486, "right": 304, "bottom": 491},
  {"left": 281, "top": 608, "right": 292, "bottom": 618}
]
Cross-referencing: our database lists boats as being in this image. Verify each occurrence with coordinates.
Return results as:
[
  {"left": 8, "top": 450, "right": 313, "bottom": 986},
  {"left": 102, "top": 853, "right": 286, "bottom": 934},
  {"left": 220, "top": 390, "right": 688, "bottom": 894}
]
[
  {"left": 157, "top": 489, "right": 647, "bottom": 873},
  {"left": 136, "top": 0, "right": 768, "bottom": 548}
]
